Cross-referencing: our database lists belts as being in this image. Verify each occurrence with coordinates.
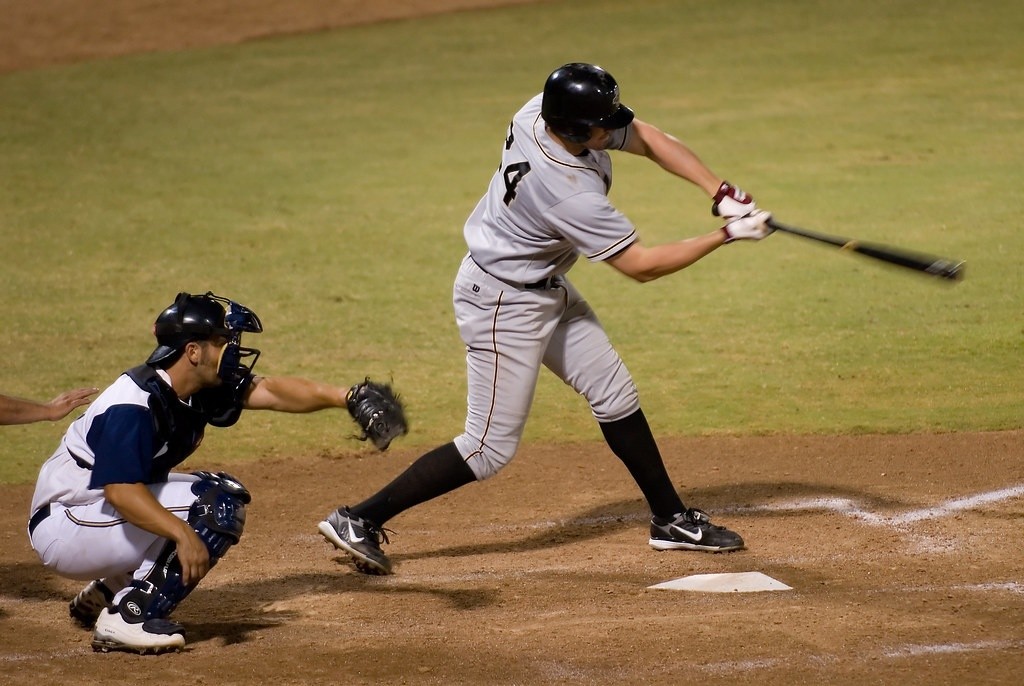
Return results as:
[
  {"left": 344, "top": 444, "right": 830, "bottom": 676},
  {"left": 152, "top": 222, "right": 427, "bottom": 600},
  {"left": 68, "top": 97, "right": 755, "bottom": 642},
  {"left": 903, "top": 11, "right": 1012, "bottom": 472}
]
[
  {"left": 29, "top": 504, "right": 50, "bottom": 537},
  {"left": 524, "top": 277, "right": 556, "bottom": 289}
]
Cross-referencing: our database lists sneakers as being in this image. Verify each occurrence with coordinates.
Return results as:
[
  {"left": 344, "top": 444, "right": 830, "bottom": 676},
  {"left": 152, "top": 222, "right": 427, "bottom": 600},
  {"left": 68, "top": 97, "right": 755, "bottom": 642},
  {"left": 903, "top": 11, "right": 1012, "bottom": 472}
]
[
  {"left": 90, "top": 607, "right": 185, "bottom": 655},
  {"left": 317, "top": 505, "right": 397, "bottom": 575},
  {"left": 648, "top": 507, "right": 744, "bottom": 554},
  {"left": 69, "top": 579, "right": 112, "bottom": 626}
]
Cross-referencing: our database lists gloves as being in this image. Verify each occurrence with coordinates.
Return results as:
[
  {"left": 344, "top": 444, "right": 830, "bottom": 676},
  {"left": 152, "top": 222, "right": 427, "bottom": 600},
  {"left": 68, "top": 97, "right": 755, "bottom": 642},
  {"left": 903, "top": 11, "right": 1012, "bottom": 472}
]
[
  {"left": 720, "top": 209, "right": 776, "bottom": 244},
  {"left": 713, "top": 181, "right": 757, "bottom": 222}
]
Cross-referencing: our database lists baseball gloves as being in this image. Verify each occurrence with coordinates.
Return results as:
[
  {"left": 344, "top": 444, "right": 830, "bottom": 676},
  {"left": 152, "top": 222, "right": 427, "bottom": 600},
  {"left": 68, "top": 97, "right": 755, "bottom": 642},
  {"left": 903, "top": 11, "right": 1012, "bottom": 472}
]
[{"left": 343, "top": 380, "right": 411, "bottom": 455}]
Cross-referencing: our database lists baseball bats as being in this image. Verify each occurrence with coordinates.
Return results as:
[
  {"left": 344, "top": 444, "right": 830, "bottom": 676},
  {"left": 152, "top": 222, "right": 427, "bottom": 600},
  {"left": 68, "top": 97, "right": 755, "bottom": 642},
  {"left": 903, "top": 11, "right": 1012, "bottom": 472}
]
[{"left": 759, "top": 215, "right": 968, "bottom": 284}]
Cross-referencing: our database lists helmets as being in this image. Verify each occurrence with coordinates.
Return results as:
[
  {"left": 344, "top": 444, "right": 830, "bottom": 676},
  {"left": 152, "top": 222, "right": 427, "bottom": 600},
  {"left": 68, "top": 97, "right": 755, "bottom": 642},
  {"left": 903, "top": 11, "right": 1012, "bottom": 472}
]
[
  {"left": 145, "top": 296, "right": 226, "bottom": 364},
  {"left": 541, "top": 62, "right": 634, "bottom": 144}
]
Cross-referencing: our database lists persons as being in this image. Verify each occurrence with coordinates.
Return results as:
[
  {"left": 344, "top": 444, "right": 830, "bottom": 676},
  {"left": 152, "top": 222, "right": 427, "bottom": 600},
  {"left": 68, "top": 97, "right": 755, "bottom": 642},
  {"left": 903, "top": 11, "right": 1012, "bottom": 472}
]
[
  {"left": 0, "top": 388, "right": 99, "bottom": 426},
  {"left": 27, "top": 293, "right": 408, "bottom": 656},
  {"left": 316, "top": 63, "right": 772, "bottom": 576}
]
